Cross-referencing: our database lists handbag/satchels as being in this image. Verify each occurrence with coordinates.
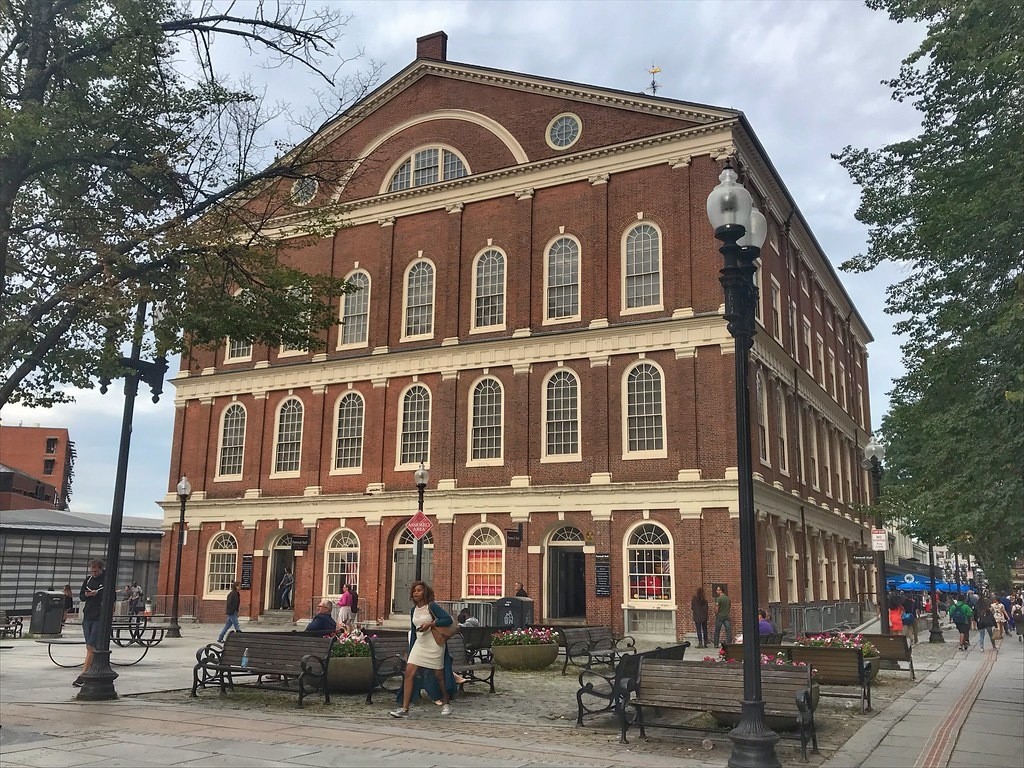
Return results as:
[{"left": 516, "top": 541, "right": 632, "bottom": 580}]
[
  {"left": 992, "top": 628, "right": 1002, "bottom": 639},
  {"left": 428, "top": 602, "right": 459, "bottom": 645},
  {"left": 902, "top": 613, "right": 915, "bottom": 625}
]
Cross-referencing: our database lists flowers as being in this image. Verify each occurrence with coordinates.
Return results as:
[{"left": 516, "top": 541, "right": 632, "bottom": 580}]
[
  {"left": 323, "top": 630, "right": 377, "bottom": 656},
  {"left": 701, "top": 651, "right": 817, "bottom": 684},
  {"left": 490, "top": 627, "right": 558, "bottom": 645},
  {"left": 800, "top": 633, "right": 879, "bottom": 656}
]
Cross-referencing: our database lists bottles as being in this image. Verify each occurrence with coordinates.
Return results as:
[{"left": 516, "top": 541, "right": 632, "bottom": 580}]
[{"left": 241, "top": 648, "right": 249, "bottom": 667}]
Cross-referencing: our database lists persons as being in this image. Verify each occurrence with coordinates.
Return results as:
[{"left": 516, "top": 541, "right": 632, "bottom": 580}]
[
  {"left": 515, "top": 582, "right": 527, "bottom": 597},
  {"left": 122, "top": 581, "right": 143, "bottom": 621},
  {"left": 922, "top": 586, "right": 1024, "bottom": 641},
  {"left": 758, "top": 608, "right": 776, "bottom": 633},
  {"left": 692, "top": 588, "right": 708, "bottom": 648},
  {"left": 337, "top": 584, "right": 358, "bottom": 629},
  {"left": 217, "top": 582, "right": 242, "bottom": 643},
  {"left": 949, "top": 595, "right": 974, "bottom": 651},
  {"left": 714, "top": 585, "right": 732, "bottom": 647},
  {"left": 303, "top": 600, "right": 336, "bottom": 632},
  {"left": 278, "top": 568, "right": 295, "bottom": 609},
  {"left": 973, "top": 598, "right": 999, "bottom": 652},
  {"left": 889, "top": 594, "right": 919, "bottom": 645},
  {"left": 389, "top": 581, "right": 471, "bottom": 718},
  {"left": 49, "top": 585, "right": 73, "bottom": 625},
  {"left": 458, "top": 608, "right": 479, "bottom": 628},
  {"left": 72, "top": 558, "right": 117, "bottom": 686}
]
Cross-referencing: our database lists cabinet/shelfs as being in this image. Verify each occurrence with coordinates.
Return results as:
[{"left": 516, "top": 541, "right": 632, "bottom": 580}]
[{"left": 0, "top": 628, "right": 13, "bottom": 639}]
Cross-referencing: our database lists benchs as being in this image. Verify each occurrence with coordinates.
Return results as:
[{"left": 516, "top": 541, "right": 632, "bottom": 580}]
[
  {"left": 719, "top": 642, "right": 873, "bottom": 715},
  {"left": 562, "top": 624, "right": 636, "bottom": 675},
  {"left": 363, "top": 632, "right": 496, "bottom": 701},
  {"left": 190, "top": 629, "right": 337, "bottom": 708},
  {"left": 576, "top": 640, "right": 691, "bottom": 727},
  {"left": 805, "top": 631, "right": 916, "bottom": 679},
  {"left": 620, "top": 655, "right": 819, "bottom": 764},
  {"left": 34, "top": 626, "right": 182, "bottom": 667},
  {"left": 203, "top": 629, "right": 337, "bottom": 688}
]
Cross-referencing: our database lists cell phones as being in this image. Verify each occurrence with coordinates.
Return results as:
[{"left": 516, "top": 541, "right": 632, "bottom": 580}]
[{"left": 416, "top": 626, "right": 422, "bottom": 632}]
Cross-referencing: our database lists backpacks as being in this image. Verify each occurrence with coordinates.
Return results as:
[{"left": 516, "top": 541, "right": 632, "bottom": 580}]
[
  {"left": 1013, "top": 605, "right": 1023, "bottom": 621},
  {"left": 975, "top": 606, "right": 991, "bottom": 624},
  {"left": 952, "top": 604, "right": 965, "bottom": 624}
]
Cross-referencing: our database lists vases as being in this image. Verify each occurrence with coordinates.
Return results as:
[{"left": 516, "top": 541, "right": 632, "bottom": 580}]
[
  {"left": 863, "top": 654, "right": 879, "bottom": 679},
  {"left": 492, "top": 644, "right": 559, "bottom": 671},
  {"left": 327, "top": 655, "right": 374, "bottom": 696},
  {"left": 712, "top": 681, "right": 818, "bottom": 732}
]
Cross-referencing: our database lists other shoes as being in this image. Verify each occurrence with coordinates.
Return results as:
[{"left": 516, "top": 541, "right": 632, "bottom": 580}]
[
  {"left": 993, "top": 646, "right": 999, "bottom": 650},
  {"left": 433, "top": 700, "right": 443, "bottom": 706},
  {"left": 456, "top": 678, "right": 466, "bottom": 683},
  {"left": 959, "top": 643, "right": 967, "bottom": 651},
  {"left": 1019, "top": 636, "right": 1021, "bottom": 642},
  {"left": 981, "top": 649, "right": 984, "bottom": 652}
]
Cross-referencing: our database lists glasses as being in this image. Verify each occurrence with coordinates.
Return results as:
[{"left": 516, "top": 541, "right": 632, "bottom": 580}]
[{"left": 316, "top": 605, "right": 329, "bottom": 610}]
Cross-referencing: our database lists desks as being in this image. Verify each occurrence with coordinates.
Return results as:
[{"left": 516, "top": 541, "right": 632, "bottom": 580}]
[
  {"left": 0, "top": 624, "right": 17, "bottom": 638},
  {"left": 112, "top": 615, "right": 169, "bottom": 624},
  {"left": 62, "top": 622, "right": 143, "bottom": 626}
]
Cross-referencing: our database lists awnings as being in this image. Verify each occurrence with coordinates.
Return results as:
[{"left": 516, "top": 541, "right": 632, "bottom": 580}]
[{"left": 886, "top": 574, "right": 977, "bottom": 592}]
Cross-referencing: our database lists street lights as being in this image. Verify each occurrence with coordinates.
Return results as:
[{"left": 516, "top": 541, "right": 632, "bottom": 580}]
[
  {"left": 705, "top": 157, "right": 785, "bottom": 768},
  {"left": 165, "top": 473, "right": 192, "bottom": 638},
  {"left": 413, "top": 458, "right": 431, "bottom": 581},
  {"left": 864, "top": 435, "right": 900, "bottom": 671},
  {"left": 74, "top": 298, "right": 181, "bottom": 700}
]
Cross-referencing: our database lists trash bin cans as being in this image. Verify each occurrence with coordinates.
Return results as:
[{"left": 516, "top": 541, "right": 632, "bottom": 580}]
[
  {"left": 497, "top": 597, "right": 534, "bottom": 626},
  {"left": 29, "top": 590, "right": 65, "bottom": 634}
]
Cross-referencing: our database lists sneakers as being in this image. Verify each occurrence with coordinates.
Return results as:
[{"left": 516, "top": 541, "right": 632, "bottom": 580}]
[
  {"left": 441, "top": 704, "right": 452, "bottom": 715},
  {"left": 390, "top": 709, "right": 409, "bottom": 718},
  {"left": 72, "top": 673, "right": 86, "bottom": 687}
]
